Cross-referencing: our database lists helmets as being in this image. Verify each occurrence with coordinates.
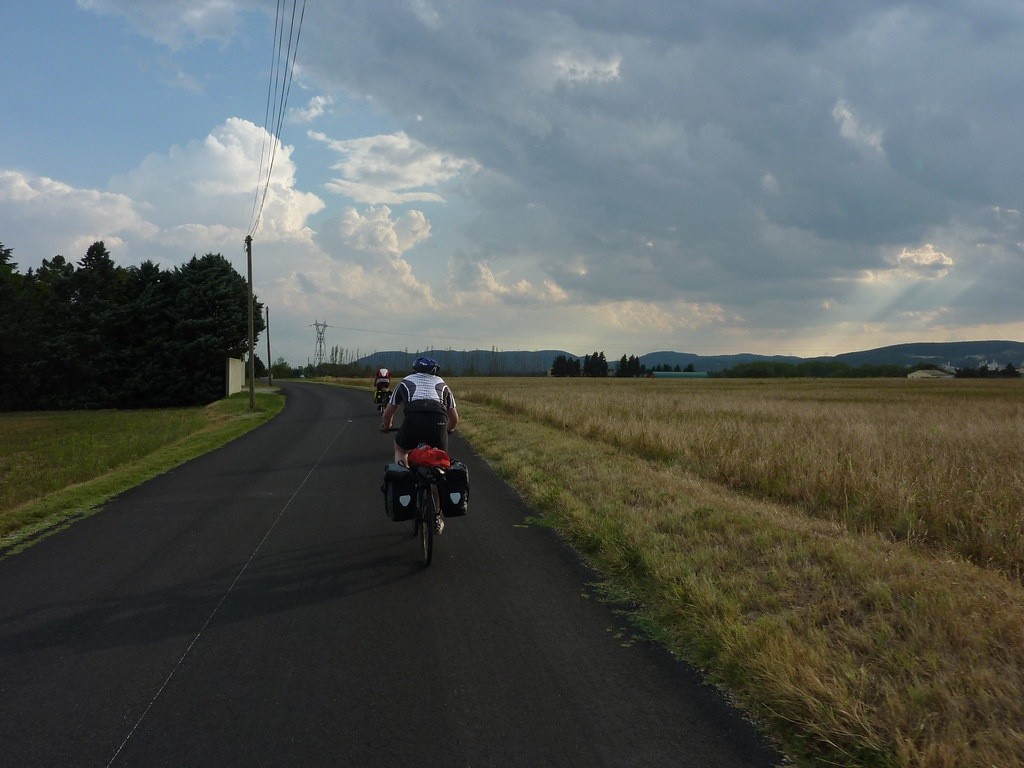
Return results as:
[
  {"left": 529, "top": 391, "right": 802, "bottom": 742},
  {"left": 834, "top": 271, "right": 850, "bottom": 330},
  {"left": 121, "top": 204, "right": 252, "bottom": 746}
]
[{"left": 412, "top": 357, "right": 440, "bottom": 373}]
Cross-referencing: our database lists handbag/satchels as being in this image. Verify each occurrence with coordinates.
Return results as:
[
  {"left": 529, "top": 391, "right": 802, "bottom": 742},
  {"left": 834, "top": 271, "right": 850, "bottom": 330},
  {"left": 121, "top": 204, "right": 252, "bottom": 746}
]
[
  {"left": 434, "top": 457, "right": 469, "bottom": 518},
  {"left": 373, "top": 390, "right": 382, "bottom": 403},
  {"left": 407, "top": 443, "right": 451, "bottom": 478},
  {"left": 380, "top": 461, "right": 418, "bottom": 522},
  {"left": 384, "top": 388, "right": 393, "bottom": 403}
]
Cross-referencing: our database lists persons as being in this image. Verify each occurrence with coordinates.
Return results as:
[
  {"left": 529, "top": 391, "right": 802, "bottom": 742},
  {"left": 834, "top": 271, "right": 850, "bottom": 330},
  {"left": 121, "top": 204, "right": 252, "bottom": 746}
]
[
  {"left": 375, "top": 367, "right": 391, "bottom": 403},
  {"left": 383, "top": 356, "right": 458, "bottom": 535}
]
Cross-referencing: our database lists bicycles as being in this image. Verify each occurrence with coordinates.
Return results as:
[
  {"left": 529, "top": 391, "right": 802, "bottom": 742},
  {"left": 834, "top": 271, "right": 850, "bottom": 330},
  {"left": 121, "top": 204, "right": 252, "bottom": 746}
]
[
  {"left": 373, "top": 383, "right": 389, "bottom": 414},
  {"left": 381, "top": 424, "right": 452, "bottom": 568}
]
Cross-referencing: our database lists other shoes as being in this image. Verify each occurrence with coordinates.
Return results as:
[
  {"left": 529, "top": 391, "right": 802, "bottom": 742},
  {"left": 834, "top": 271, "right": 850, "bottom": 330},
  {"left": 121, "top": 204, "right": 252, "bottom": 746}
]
[{"left": 434, "top": 519, "right": 444, "bottom": 536}]
